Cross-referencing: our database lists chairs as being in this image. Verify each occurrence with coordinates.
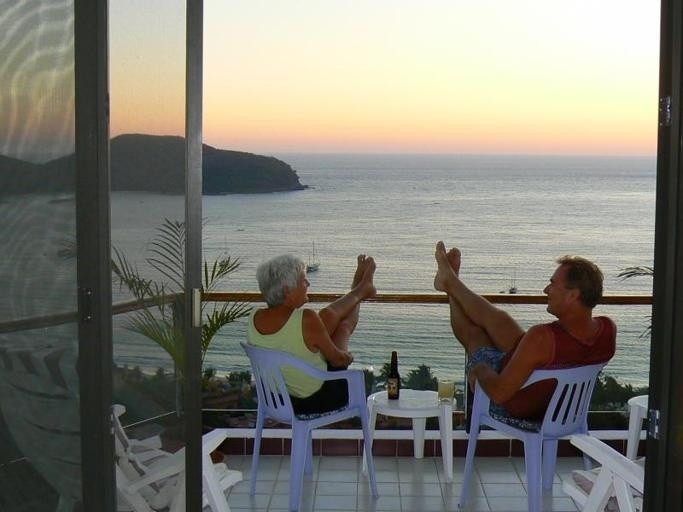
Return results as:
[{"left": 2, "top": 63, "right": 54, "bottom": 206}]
[
  {"left": 458, "top": 361, "right": 610, "bottom": 512},
  {"left": 240, "top": 340, "right": 379, "bottom": 512},
  {"left": 0, "top": 344, "right": 244, "bottom": 512},
  {"left": 562, "top": 394, "right": 645, "bottom": 512}
]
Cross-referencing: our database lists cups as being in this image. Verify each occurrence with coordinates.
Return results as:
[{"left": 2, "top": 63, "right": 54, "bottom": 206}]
[{"left": 439, "top": 378, "right": 455, "bottom": 402}]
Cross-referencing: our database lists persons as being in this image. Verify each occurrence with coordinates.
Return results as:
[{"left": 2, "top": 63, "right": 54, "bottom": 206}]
[
  {"left": 244, "top": 251, "right": 378, "bottom": 417},
  {"left": 431, "top": 238, "right": 618, "bottom": 423}
]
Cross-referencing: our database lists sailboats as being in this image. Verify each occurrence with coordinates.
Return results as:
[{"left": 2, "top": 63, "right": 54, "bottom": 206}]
[
  {"left": 217, "top": 234, "right": 231, "bottom": 270},
  {"left": 304, "top": 239, "right": 322, "bottom": 273},
  {"left": 509, "top": 270, "right": 519, "bottom": 294}
]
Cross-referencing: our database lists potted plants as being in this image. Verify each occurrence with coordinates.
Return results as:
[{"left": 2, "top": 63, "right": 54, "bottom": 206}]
[{"left": 52, "top": 216, "right": 256, "bottom": 464}]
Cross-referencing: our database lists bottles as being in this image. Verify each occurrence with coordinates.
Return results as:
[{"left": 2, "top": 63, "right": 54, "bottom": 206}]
[{"left": 387, "top": 349, "right": 401, "bottom": 399}]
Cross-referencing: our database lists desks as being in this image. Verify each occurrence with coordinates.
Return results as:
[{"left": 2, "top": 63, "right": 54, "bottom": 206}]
[
  {"left": 626, "top": 394, "right": 649, "bottom": 460},
  {"left": 362, "top": 388, "right": 458, "bottom": 484}
]
